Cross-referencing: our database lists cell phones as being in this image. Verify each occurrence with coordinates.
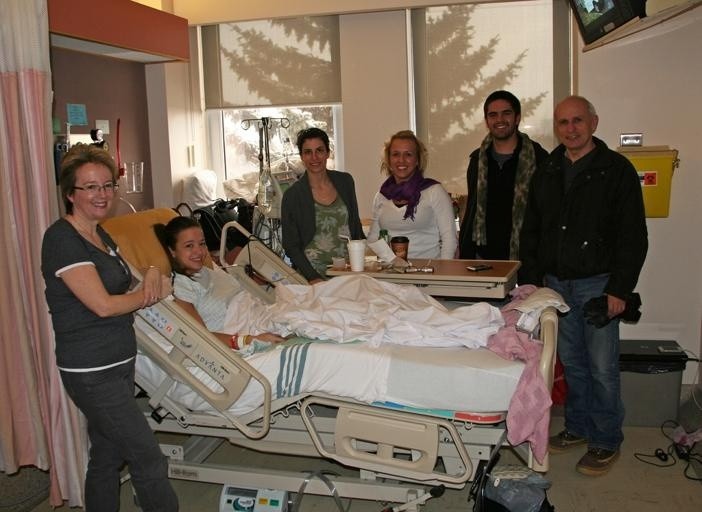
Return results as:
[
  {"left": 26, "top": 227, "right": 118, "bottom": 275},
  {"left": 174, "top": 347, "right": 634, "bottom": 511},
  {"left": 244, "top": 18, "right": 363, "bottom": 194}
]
[
  {"left": 659, "top": 346, "right": 681, "bottom": 353},
  {"left": 466, "top": 264, "right": 492, "bottom": 271}
]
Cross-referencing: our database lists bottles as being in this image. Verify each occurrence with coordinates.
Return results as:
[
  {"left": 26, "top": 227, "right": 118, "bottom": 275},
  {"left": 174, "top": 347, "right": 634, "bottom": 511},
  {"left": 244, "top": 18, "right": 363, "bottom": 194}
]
[
  {"left": 377, "top": 230, "right": 389, "bottom": 257},
  {"left": 257, "top": 166, "right": 274, "bottom": 216}
]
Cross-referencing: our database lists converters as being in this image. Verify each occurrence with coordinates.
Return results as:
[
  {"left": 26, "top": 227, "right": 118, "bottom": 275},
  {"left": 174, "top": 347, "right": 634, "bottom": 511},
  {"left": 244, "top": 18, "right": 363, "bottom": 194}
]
[{"left": 674, "top": 443, "right": 689, "bottom": 460}]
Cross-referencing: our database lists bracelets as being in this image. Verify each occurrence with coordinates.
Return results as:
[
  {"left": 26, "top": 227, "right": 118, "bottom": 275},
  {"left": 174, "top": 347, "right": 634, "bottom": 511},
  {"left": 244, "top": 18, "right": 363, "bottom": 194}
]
[
  {"left": 145, "top": 264, "right": 160, "bottom": 271},
  {"left": 229, "top": 332, "right": 252, "bottom": 351}
]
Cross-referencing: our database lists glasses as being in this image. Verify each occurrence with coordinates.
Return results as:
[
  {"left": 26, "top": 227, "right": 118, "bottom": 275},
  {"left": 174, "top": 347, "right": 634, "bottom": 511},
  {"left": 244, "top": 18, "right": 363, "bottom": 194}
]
[{"left": 74, "top": 184, "right": 119, "bottom": 192}]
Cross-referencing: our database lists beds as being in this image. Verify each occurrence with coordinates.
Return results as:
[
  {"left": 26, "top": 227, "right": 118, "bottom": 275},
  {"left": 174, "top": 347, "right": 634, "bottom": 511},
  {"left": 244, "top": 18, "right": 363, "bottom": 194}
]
[{"left": 96, "top": 201, "right": 559, "bottom": 512}]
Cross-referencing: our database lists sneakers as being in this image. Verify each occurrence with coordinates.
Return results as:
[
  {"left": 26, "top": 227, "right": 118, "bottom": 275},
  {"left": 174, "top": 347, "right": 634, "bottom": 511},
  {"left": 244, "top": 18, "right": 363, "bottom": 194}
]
[
  {"left": 576, "top": 448, "right": 621, "bottom": 477},
  {"left": 548, "top": 429, "right": 589, "bottom": 455}
]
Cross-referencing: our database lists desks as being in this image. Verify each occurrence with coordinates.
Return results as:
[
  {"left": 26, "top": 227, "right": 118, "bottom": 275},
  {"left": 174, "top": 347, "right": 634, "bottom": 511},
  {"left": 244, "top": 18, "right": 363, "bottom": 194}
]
[{"left": 326, "top": 258, "right": 522, "bottom": 298}]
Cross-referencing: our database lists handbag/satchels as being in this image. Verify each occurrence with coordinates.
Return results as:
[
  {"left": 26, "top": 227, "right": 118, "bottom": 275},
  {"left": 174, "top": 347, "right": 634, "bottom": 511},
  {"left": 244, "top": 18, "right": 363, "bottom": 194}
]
[{"left": 192, "top": 198, "right": 253, "bottom": 250}]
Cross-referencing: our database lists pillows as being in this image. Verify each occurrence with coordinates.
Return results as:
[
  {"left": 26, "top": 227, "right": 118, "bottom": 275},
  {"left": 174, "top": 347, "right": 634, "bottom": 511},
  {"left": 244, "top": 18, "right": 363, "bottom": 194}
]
[{"left": 99, "top": 208, "right": 213, "bottom": 278}]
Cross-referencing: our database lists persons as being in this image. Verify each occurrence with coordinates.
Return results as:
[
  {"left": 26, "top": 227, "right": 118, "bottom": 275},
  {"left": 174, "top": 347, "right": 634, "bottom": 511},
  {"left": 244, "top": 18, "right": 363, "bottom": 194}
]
[
  {"left": 40, "top": 143, "right": 180, "bottom": 512},
  {"left": 460, "top": 89, "right": 550, "bottom": 260},
  {"left": 281, "top": 129, "right": 366, "bottom": 286},
  {"left": 519, "top": 94, "right": 649, "bottom": 478},
  {"left": 152, "top": 214, "right": 295, "bottom": 344},
  {"left": 366, "top": 128, "right": 460, "bottom": 266}
]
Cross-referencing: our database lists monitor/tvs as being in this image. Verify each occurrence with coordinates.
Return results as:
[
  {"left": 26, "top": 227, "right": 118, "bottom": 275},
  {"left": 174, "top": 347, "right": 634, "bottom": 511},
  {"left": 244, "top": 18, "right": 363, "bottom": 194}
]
[{"left": 570, "top": 0, "right": 647, "bottom": 46}]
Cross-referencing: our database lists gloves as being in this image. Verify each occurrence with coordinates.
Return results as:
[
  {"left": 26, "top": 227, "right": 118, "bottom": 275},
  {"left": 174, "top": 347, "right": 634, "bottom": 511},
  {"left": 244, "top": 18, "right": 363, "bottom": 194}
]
[{"left": 584, "top": 293, "right": 641, "bottom": 328}]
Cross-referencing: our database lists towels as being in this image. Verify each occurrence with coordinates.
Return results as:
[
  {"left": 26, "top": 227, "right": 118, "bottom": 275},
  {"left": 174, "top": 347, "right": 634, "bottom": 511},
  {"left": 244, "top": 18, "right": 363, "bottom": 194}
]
[{"left": 514, "top": 288, "right": 571, "bottom": 332}]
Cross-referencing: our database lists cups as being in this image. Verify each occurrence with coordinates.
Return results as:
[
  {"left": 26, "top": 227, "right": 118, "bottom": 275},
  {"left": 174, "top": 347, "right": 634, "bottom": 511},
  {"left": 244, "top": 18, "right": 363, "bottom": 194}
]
[
  {"left": 125, "top": 162, "right": 145, "bottom": 193},
  {"left": 346, "top": 240, "right": 365, "bottom": 272},
  {"left": 390, "top": 235, "right": 409, "bottom": 260}
]
